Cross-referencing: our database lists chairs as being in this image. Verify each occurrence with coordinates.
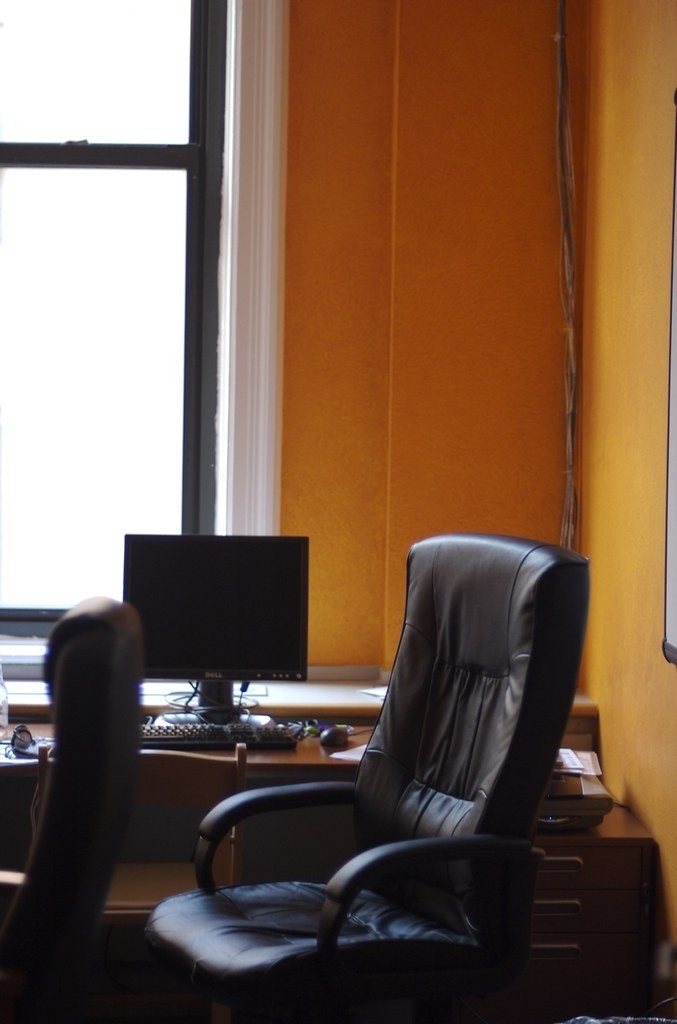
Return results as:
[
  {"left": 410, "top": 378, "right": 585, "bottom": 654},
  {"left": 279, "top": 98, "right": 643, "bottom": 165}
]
[
  {"left": 142, "top": 531, "right": 590, "bottom": 1023},
  {"left": 0, "top": 596, "right": 135, "bottom": 1024}
]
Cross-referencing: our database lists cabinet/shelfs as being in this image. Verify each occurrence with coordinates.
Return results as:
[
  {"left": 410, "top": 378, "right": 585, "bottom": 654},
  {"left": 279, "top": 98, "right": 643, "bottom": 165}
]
[{"left": 451, "top": 805, "right": 657, "bottom": 1024}]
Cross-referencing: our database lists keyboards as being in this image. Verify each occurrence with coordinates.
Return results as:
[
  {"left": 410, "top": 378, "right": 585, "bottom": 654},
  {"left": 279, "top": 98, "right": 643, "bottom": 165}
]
[{"left": 137, "top": 723, "right": 299, "bottom": 749}]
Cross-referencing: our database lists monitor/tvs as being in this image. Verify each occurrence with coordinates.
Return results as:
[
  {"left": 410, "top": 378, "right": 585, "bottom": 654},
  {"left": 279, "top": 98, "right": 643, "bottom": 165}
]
[{"left": 122, "top": 532, "right": 310, "bottom": 726}]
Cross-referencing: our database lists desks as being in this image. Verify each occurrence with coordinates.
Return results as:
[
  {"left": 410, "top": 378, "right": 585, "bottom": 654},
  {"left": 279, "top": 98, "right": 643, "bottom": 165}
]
[{"left": 0, "top": 679, "right": 595, "bottom": 1023}]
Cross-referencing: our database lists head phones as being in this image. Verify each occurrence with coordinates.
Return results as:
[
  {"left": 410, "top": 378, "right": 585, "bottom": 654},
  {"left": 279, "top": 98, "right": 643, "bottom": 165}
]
[{"left": 11, "top": 725, "right": 57, "bottom": 758}]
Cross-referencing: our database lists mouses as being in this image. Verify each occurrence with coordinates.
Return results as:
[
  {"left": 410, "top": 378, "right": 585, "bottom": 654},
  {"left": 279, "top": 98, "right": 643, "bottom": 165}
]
[{"left": 320, "top": 726, "right": 351, "bottom": 746}]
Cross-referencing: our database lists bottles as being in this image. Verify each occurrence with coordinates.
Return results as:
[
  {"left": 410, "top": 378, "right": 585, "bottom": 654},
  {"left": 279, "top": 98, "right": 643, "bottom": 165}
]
[{"left": 0, "top": 660, "right": 9, "bottom": 737}]
